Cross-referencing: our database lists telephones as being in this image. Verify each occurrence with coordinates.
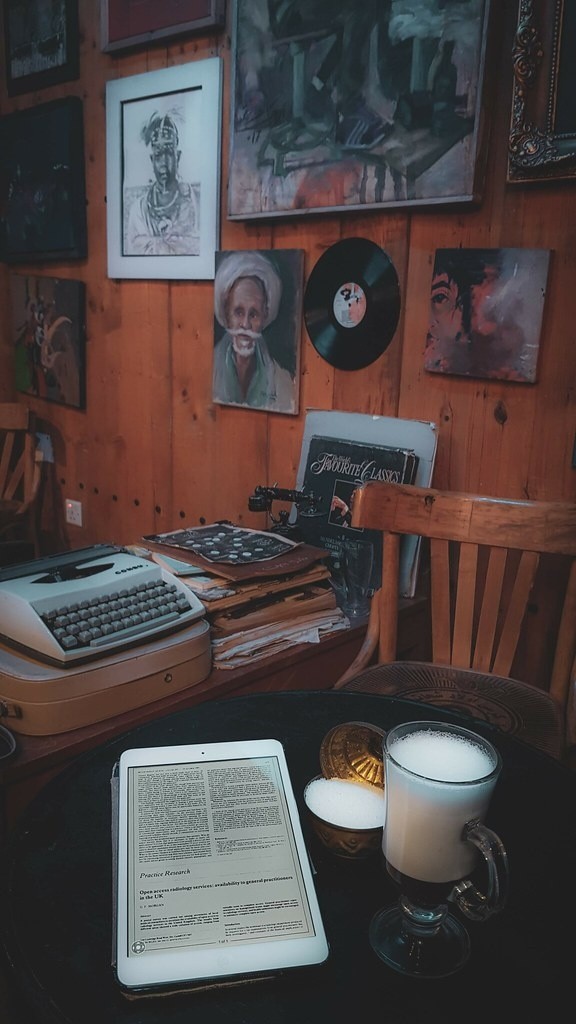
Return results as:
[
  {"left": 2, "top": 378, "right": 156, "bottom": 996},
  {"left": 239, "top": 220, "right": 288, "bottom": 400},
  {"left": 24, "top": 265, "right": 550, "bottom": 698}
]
[{"left": 247, "top": 485, "right": 328, "bottom": 545}]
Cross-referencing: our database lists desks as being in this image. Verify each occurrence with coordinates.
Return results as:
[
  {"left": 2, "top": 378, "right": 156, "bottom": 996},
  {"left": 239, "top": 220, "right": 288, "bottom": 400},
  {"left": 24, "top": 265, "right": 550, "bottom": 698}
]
[{"left": 0, "top": 690, "right": 576, "bottom": 1024}]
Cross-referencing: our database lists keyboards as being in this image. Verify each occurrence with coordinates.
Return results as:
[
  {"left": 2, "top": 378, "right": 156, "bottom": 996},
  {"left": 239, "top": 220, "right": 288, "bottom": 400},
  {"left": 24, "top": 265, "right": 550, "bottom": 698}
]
[{"left": 42, "top": 576, "right": 190, "bottom": 648}]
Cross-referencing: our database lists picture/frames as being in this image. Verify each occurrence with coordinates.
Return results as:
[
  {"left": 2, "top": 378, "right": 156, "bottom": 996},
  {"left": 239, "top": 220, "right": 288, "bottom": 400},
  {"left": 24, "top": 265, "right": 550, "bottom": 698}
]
[
  {"left": 3, "top": 0, "right": 80, "bottom": 98},
  {"left": 503, "top": 0, "right": 576, "bottom": 193},
  {"left": 99, "top": 0, "right": 226, "bottom": 55},
  {"left": 225, "top": 0, "right": 498, "bottom": 222},
  {"left": 106, "top": 56, "right": 224, "bottom": 287},
  {"left": 0, "top": 93, "right": 88, "bottom": 266}
]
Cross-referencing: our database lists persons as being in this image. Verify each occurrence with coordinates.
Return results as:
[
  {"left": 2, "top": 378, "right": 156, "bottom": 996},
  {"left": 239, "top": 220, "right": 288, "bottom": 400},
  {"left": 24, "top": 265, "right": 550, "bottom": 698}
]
[{"left": 330, "top": 487, "right": 360, "bottom": 528}]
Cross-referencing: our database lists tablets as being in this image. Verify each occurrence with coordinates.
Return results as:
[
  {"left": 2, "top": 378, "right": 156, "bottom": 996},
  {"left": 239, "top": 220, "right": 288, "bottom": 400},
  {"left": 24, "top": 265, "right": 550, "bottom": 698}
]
[{"left": 116, "top": 740, "right": 328, "bottom": 985}]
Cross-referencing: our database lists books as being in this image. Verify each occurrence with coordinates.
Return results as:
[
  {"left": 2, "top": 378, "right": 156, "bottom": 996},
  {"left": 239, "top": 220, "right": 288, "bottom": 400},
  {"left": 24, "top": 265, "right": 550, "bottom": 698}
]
[
  {"left": 122, "top": 540, "right": 350, "bottom": 669},
  {"left": 288, "top": 410, "right": 438, "bottom": 596}
]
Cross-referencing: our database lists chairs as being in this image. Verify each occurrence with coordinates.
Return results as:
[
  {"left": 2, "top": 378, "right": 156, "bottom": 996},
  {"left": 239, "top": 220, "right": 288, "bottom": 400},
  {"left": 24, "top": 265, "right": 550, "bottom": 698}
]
[
  {"left": 0, "top": 401, "right": 44, "bottom": 558},
  {"left": 331, "top": 478, "right": 576, "bottom": 769}
]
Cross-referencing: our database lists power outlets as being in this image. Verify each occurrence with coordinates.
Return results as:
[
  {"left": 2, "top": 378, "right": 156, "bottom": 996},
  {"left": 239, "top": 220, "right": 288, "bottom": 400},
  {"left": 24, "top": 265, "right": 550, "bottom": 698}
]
[{"left": 65, "top": 498, "right": 83, "bottom": 527}]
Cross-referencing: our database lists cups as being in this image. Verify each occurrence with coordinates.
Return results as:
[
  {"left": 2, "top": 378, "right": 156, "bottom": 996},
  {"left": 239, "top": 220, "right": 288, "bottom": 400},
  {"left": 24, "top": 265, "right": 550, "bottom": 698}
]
[
  {"left": 304, "top": 772, "right": 383, "bottom": 858},
  {"left": 384, "top": 722, "right": 506, "bottom": 921}
]
